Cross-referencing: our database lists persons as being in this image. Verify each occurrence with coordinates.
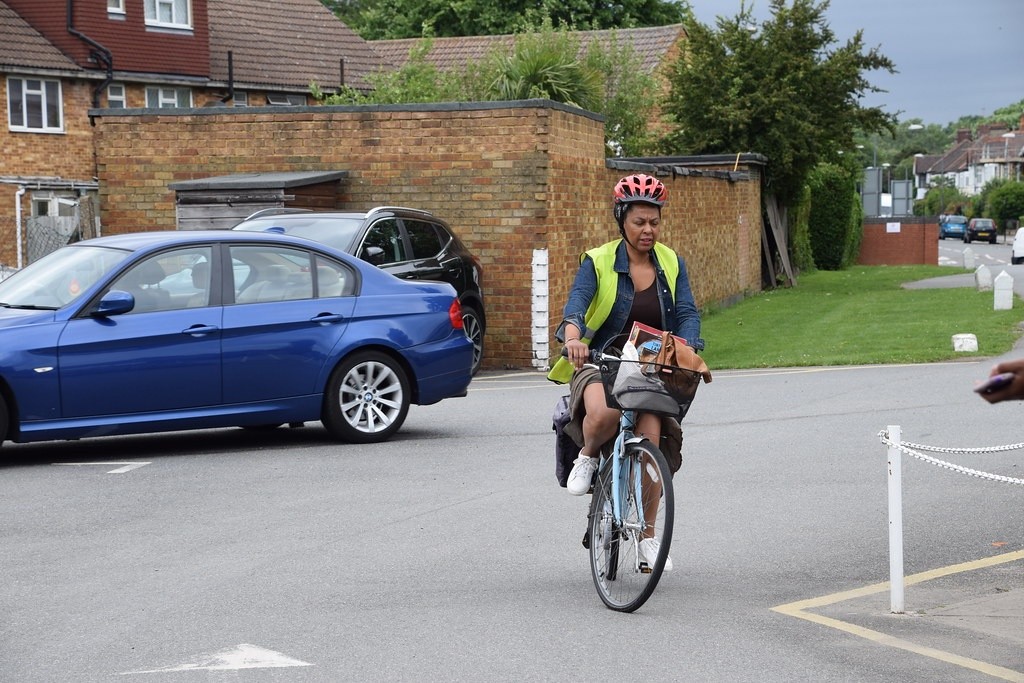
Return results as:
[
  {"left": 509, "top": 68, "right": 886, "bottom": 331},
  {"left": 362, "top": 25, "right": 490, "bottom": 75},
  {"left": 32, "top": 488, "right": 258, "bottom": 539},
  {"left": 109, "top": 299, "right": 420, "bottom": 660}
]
[
  {"left": 973, "top": 357, "right": 1024, "bottom": 404},
  {"left": 548, "top": 173, "right": 705, "bottom": 572}
]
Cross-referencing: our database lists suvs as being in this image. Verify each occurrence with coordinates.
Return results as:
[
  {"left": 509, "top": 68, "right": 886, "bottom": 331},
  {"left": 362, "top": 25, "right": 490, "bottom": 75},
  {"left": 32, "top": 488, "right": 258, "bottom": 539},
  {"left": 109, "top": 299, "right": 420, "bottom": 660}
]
[{"left": 148, "top": 205, "right": 487, "bottom": 379}]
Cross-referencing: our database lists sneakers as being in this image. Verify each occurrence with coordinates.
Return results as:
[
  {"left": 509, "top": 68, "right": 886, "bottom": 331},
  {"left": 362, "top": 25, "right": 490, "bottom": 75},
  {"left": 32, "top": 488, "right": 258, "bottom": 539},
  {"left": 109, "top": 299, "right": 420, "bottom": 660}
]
[
  {"left": 566, "top": 447, "right": 598, "bottom": 496},
  {"left": 634, "top": 535, "right": 673, "bottom": 572}
]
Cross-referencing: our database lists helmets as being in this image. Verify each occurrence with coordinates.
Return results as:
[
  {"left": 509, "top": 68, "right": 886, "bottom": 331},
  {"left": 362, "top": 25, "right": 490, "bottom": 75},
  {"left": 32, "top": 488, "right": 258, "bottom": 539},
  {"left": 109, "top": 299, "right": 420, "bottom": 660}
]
[{"left": 613, "top": 174, "right": 668, "bottom": 219}]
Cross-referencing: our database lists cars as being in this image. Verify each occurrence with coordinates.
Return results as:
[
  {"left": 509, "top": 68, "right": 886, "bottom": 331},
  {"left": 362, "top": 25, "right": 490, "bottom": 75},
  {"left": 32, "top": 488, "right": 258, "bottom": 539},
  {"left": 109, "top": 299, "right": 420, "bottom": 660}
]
[
  {"left": 0, "top": 229, "right": 475, "bottom": 454},
  {"left": 938, "top": 215, "right": 968, "bottom": 240},
  {"left": 1011, "top": 227, "right": 1024, "bottom": 264},
  {"left": 962, "top": 217, "right": 997, "bottom": 245}
]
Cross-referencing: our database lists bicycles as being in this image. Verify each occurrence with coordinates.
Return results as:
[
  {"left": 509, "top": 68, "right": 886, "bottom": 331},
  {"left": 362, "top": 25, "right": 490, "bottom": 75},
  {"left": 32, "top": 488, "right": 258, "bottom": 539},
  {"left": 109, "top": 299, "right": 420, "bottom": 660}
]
[{"left": 560, "top": 333, "right": 703, "bottom": 614}]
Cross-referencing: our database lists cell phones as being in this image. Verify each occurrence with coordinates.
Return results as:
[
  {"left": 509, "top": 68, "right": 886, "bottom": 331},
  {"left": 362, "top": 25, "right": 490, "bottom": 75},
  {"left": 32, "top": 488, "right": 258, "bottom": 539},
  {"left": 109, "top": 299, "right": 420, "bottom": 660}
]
[{"left": 974, "top": 371, "right": 1015, "bottom": 395}]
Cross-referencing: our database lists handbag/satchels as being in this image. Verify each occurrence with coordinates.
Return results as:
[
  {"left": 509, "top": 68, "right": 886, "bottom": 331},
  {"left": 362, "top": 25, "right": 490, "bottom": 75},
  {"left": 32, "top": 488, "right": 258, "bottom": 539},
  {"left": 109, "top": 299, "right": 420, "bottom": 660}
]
[
  {"left": 552, "top": 394, "right": 597, "bottom": 488},
  {"left": 611, "top": 330, "right": 712, "bottom": 414}
]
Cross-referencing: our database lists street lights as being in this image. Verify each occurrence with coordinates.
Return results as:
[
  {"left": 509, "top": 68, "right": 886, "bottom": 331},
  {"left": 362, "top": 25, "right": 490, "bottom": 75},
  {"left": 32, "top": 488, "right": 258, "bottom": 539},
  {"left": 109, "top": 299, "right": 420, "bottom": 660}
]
[
  {"left": 883, "top": 163, "right": 909, "bottom": 215},
  {"left": 911, "top": 153, "right": 923, "bottom": 175}
]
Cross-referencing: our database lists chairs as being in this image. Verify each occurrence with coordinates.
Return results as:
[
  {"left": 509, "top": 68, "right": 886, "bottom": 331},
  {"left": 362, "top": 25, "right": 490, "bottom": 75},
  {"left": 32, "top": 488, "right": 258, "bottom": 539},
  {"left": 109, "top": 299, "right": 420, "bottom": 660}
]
[
  {"left": 184, "top": 261, "right": 211, "bottom": 307},
  {"left": 237, "top": 264, "right": 345, "bottom": 303},
  {"left": 135, "top": 261, "right": 169, "bottom": 304}
]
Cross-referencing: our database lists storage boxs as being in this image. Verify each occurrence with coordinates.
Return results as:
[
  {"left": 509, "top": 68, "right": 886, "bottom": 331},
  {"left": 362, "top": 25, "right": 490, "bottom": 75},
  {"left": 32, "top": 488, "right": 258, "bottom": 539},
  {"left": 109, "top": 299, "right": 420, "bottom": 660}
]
[{"left": 628, "top": 321, "right": 687, "bottom": 350}]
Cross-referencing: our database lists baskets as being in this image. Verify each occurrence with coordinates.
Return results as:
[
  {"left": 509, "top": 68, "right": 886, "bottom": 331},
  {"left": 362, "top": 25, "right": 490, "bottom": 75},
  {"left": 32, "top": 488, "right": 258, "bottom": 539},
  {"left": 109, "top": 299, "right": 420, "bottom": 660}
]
[{"left": 599, "top": 359, "right": 701, "bottom": 418}]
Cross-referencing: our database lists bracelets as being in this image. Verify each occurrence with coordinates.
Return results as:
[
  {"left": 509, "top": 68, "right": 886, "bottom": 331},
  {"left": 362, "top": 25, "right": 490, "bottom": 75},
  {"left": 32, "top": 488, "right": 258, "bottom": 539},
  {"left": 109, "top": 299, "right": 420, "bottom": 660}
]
[{"left": 565, "top": 337, "right": 579, "bottom": 344}]
